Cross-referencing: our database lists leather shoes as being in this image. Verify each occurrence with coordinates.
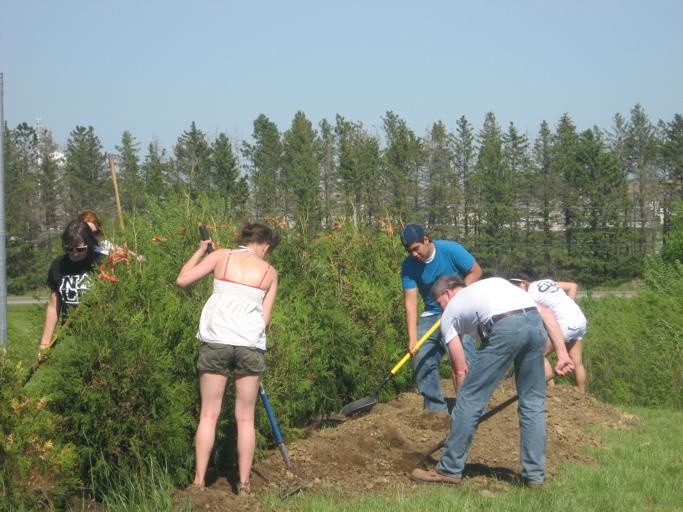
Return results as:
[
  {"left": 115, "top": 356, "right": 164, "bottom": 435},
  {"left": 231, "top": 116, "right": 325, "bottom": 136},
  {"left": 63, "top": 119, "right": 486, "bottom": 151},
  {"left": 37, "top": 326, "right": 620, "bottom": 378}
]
[
  {"left": 511, "top": 472, "right": 544, "bottom": 490},
  {"left": 410, "top": 468, "right": 461, "bottom": 486}
]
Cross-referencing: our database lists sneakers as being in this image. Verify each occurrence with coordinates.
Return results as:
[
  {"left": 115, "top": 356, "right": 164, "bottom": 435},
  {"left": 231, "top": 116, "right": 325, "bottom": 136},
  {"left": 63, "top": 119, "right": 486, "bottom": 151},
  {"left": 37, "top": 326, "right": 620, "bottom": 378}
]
[{"left": 190, "top": 481, "right": 252, "bottom": 496}]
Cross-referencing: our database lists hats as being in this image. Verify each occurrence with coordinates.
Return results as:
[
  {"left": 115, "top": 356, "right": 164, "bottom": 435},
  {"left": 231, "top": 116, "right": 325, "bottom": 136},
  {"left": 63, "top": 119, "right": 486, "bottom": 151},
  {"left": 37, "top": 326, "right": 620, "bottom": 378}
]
[{"left": 399, "top": 223, "right": 429, "bottom": 249}]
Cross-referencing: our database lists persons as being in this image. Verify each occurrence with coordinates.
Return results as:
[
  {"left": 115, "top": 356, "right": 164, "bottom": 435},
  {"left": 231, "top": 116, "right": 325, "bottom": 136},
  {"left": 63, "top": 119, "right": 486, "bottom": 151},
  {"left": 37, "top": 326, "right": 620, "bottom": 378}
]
[
  {"left": 400, "top": 224, "right": 483, "bottom": 412},
  {"left": 408, "top": 273, "right": 575, "bottom": 491},
  {"left": 507, "top": 270, "right": 590, "bottom": 395},
  {"left": 174, "top": 222, "right": 281, "bottom": 498},
  {"left": 76, "top": 209, "right": 149, "bottom": 265},
  {"left": 36, "top": 217, "right": 111, "bottom": 366}
]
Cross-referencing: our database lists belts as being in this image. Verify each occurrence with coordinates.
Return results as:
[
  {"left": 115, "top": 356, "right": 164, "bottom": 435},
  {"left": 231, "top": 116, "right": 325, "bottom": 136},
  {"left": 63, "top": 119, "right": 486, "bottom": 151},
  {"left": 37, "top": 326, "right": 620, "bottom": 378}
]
[{"left": 482, "top": 306, "right": 538, "bottom": 332}]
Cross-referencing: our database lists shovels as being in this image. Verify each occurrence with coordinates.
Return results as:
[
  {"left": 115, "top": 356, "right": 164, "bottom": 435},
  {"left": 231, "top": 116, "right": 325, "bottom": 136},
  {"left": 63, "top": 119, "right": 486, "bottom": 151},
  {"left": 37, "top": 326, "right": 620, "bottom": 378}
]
[
  {"left": 401, "top": 363, "right": 570, "bottom": 473},
  {"left": 340, "top": 318, "right": 441, "bottom": 416}
]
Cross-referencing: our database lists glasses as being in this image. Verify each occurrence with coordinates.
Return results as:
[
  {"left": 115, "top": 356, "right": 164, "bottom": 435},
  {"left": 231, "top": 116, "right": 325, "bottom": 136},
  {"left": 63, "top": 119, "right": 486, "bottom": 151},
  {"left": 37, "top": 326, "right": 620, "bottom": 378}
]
[{"left": 63, "top": 245, "right": 88, "bottom": 254}]
